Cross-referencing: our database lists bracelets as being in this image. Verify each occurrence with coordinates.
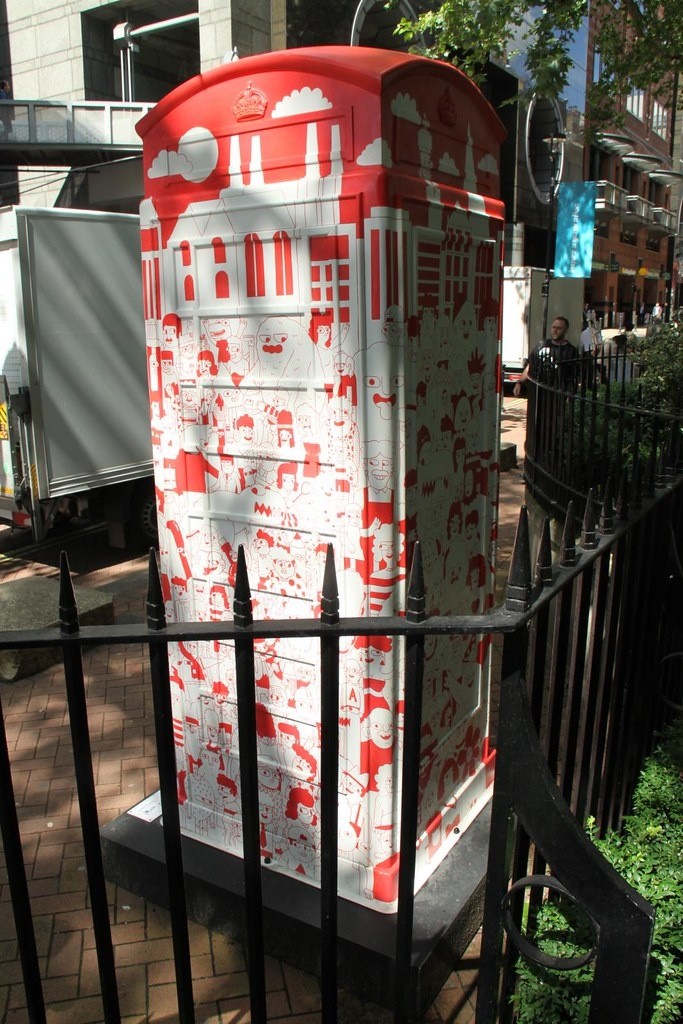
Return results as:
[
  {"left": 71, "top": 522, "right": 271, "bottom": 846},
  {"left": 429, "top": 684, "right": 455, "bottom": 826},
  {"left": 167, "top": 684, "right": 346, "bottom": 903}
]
[{"left": 515, "top": 380, "right": 523, "bottom": 386}]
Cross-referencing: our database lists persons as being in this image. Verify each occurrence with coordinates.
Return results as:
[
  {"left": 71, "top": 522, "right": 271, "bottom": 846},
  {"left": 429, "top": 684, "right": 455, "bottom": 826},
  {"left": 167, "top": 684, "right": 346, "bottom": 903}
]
[
  {"left": 514, "top": 317, "right": 581, "bottom": 477},
  {"left": 580, "top": 302, "right": 665, "bottom": 386}
]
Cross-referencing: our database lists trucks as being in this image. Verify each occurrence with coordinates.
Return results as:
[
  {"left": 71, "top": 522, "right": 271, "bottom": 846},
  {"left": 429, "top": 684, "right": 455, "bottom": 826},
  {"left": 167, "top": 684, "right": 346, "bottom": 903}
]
[
  {"left": 499, "top": 265, "right": 585, "bottom": 391},
  {"left": 1, "top": 203, "right": 160, "bottom": 553}
]
